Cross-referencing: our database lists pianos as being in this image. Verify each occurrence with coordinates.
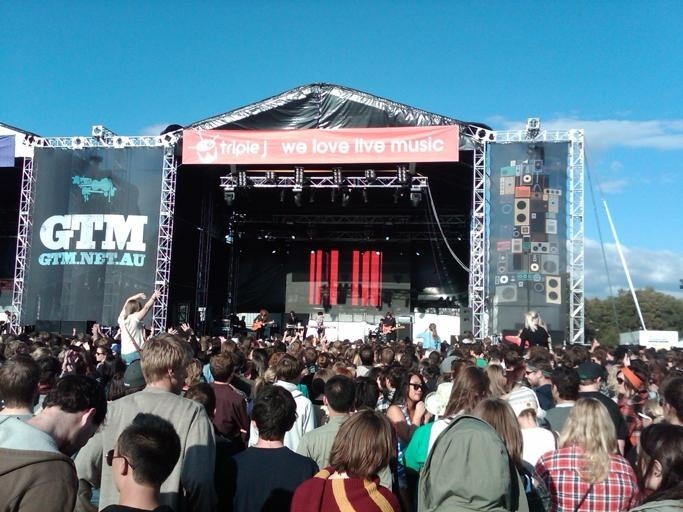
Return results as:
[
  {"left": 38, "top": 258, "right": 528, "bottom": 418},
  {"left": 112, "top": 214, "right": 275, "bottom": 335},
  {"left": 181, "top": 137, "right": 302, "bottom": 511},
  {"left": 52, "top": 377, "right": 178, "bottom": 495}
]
[{"left": 286, "top": 324, "right": 304, "bottom": 330}]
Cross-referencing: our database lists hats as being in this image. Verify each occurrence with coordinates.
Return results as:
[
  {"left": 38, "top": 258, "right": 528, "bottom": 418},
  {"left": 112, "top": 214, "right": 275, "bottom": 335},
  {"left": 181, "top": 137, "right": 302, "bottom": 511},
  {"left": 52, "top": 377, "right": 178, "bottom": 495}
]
[
  {"left": 110, "top": 344, "right": 120, "bottom": 353},
  {"left": 577, "top": 361, "right": 609, "bottom": 380},
  {"left": 440, "top": 356, "right": 461, "bottom": 374},
  {"left": 424, "top": 382, "right": 454, "bottom": 417}
]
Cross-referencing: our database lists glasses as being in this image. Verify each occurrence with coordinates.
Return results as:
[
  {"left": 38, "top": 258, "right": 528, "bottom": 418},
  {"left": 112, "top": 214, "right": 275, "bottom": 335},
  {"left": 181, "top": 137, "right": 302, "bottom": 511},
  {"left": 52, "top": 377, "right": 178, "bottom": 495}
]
[
  {"left": 106, "top": 449, "right": 135, "bottom": 471},
  {"left": 616, "top": 377, "right": 624, "bottom": 384},
  {"left": 96, "top": 352, "right": 102, "bottom": 355},
  {"left": 409, "top": 382, "right": 426, "bottom": 392},
  {"left": 526, "top": 371, "right": 533, "bottom": 374}
]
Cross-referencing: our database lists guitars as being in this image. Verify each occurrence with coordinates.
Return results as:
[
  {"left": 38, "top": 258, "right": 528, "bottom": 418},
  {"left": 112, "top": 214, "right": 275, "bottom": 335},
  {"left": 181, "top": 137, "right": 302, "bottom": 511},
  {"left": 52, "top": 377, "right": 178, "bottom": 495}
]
[
  {"left": 251, "top": 320, "right": 275, "bottom": 330},
  {"left": 383, "top": 324, "right": 405, "bottom": 335}
]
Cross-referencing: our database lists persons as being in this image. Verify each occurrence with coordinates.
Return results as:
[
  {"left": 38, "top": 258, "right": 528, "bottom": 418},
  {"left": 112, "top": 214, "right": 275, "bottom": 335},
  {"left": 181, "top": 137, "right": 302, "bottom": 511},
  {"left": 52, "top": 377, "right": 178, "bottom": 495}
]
[{"left": 0, "top": 290, "right": 683, "bottom": 512}]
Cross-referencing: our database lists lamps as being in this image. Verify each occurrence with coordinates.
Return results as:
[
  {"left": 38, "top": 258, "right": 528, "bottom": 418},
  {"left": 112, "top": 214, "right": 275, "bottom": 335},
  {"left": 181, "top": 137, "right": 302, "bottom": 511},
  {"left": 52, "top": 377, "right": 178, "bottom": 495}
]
[{"left": 220, "top": 165, "right": 422, "bottom": 206}]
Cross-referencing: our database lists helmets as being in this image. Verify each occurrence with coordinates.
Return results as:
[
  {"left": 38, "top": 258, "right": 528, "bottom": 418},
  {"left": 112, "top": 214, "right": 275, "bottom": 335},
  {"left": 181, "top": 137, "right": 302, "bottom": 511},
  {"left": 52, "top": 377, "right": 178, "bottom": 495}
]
[{"left": 507, "top": 388, "right": 538, "bottom": 419}]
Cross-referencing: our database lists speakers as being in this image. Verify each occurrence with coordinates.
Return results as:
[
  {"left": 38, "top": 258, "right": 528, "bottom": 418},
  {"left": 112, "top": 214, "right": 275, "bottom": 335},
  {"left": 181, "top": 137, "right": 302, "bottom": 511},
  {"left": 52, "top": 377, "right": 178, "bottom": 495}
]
[
  {"left": 36, "top": 318, "right": 60, "bottom": 335},
  {"left": 450, "top": 334, "right": 462, "bottom": 344},
  {"left": 397, "top": 323, "right": 413, "bottom": 343},
  {"left": 303, "top": 335, "right": 313, "bottom": 344},
  {"left": 61, "top": 320, "right": 97, "bottom": 336},
  {"left": 460, "top": 307, "right": 473, "bottom": 334},
  {"left": 496, "top": 158, "right": 565, "bottom": 346}
]
